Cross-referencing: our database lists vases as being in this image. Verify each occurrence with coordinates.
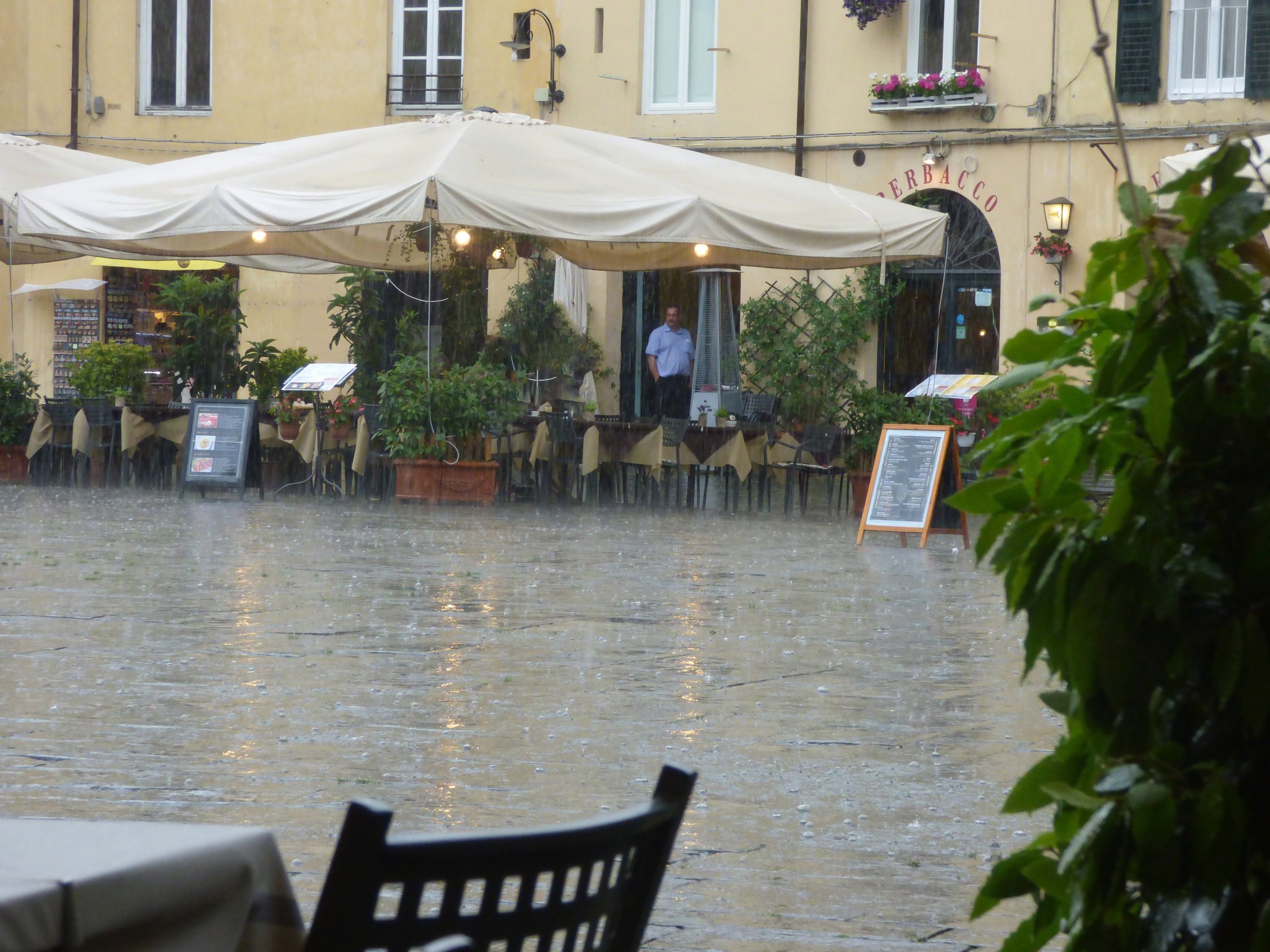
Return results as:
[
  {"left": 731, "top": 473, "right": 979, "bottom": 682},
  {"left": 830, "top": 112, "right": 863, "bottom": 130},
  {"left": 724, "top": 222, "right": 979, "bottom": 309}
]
[
  {"left": 329, "top": 423, "right": 350, "bottom": 440},
  {"left": 698, "top": 416, "right": 707, "bottom": 426},
  {"left": 279, "top": 423, "right": 301, "bottom": 440},
  {"left": 1042, "top": 252, "right": 1064, "bottom": 264}
]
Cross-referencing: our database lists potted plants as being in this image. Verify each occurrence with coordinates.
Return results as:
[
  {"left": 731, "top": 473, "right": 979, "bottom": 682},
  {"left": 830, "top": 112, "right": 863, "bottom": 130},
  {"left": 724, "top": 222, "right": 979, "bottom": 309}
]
[
  {"left": 372, "top": 356, "right": 521, "bottom": 507},
  {"left": 713, "top": 404, "right": 728, "bottom": 427},
  {"left": 0, "top": 352, "right": 41, "bottom": 484},
  {"left": 111, "top": 386, "right": 130, "bottom": 407},
  {"left": 836, "top": 381, "right": 892, "bottom": 517},
  {"left": 584, "top": 400, "right": 597, "bottom": 421}
]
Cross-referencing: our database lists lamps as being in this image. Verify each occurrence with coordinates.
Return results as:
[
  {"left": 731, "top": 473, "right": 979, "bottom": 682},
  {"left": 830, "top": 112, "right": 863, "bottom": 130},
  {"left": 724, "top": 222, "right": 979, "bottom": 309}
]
[
  {"left": 497, "top": 9, "right": 567, "bottom": 116},
  {"left": 1039, "top": 197, "right": 1073, "bottom": 232}
]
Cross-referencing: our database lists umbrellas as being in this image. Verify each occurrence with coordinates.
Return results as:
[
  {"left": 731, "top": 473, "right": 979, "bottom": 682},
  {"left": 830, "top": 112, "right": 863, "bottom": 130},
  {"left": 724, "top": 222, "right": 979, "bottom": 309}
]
[{"left": 552, "top": 249, "right": 600, "bottom": 423}]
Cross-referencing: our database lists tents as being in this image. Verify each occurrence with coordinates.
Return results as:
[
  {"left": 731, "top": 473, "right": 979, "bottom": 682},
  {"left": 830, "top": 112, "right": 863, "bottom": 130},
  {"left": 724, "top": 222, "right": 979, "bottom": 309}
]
[{"left": 0, "top": 105, "right": 950, "bottom": 425}]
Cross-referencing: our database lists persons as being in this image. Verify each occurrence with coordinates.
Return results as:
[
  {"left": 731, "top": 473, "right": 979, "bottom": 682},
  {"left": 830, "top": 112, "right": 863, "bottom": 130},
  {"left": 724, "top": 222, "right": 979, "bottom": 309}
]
[{"left": 645, "top": 303, "right": 696, "bottom": 423}]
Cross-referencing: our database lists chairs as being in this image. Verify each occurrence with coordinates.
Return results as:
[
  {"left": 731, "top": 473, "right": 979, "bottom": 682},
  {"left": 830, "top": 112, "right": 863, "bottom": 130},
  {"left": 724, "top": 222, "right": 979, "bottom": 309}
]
[
  {"left": 26, "top": 395, "right": 153, "bottom": 491},
  {"left": 493, "top": 415, "right": 840, "bottom": 517},
  {"left": 687, "top": 424, "right": 795, "bottom": 515}
]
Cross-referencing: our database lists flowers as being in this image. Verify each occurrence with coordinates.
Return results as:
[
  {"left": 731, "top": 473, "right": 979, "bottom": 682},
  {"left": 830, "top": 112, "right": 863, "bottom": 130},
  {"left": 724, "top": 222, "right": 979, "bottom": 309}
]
[
  {"left": 268, "top": 391, "right": 304, "bottom": 422},
  {"left": 1031, "top": 233, "right": 1074, "bottom": 256},
  {"left": 696, "top": 400, "right": 712, "bottom": 415},
  {"left": 323, "top": 397, "right": 365, "bottom": 424}
]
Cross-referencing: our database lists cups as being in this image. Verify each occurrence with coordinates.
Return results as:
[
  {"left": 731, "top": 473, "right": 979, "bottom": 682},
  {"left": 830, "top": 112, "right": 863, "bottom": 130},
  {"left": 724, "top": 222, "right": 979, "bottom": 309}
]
[
  {"left": 269, "top": 398, "right": 280, "bottom": 409},
  {"left": 545, "top": 406, "right": 552, "bottom": 412},
  {"left": 563, "top": 410, "right": 574, "bottom": 419},
  {"left": 531, "top": 411, "right": 539, "bottom": 416}
]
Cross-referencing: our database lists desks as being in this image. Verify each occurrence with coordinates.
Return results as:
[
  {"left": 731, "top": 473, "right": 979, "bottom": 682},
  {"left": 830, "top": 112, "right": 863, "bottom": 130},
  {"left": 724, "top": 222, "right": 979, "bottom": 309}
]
[
  {"left": 109, "top": 406, "right": 190, "bottom": 490},
  {"left": 516, "top": 416, "right": 656, "bottom": 506}
]
